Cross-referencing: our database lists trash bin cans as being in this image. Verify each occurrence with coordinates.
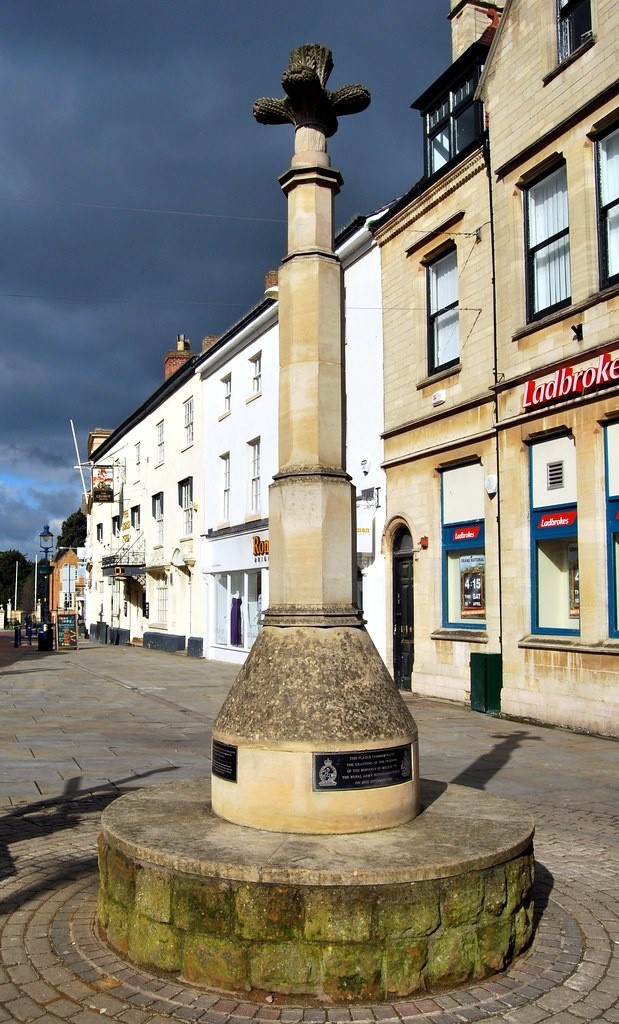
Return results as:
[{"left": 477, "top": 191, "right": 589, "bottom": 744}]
[
  {"left": 469, "top": 651, "right": 502, "bottom": 713},
  {"left": 37, "top": 622, "right": 53, "bottom": 651}
]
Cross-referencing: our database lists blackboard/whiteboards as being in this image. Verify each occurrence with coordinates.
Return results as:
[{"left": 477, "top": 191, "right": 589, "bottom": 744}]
[{"left": 55, "top": 613, "right": 78, "bottom": 648}]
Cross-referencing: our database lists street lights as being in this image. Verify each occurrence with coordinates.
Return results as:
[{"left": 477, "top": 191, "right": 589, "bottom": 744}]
[{"left": 38, "top": 525, "right": 56, "bottom": 650}]
